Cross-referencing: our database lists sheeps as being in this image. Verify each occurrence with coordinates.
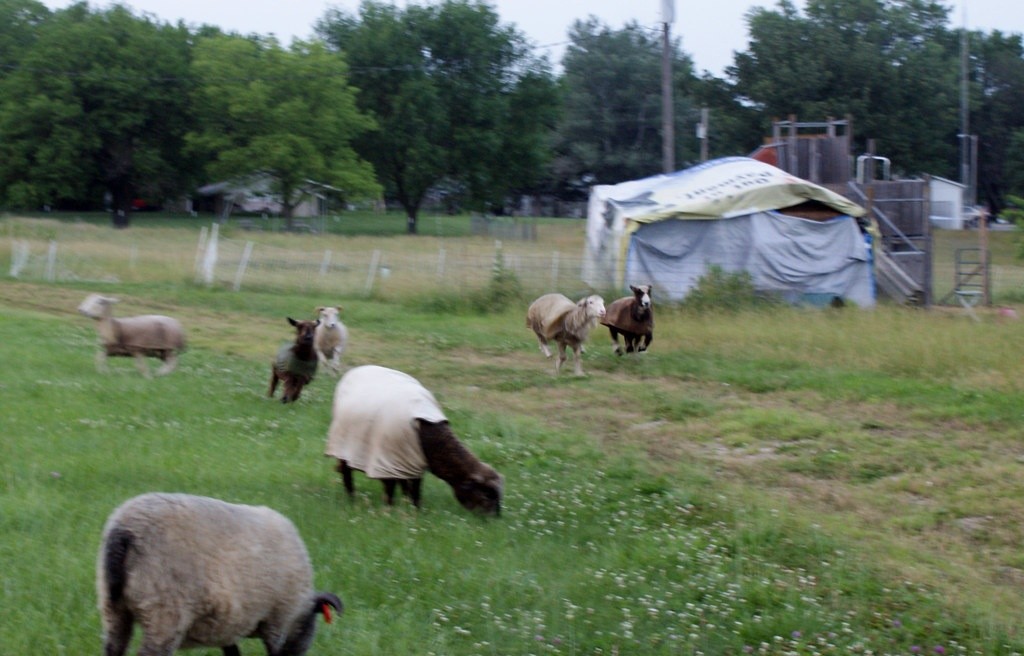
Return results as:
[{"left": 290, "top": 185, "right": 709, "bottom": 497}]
[
  {"left": 323, "top": 365, "right": 505, "bottom": 519},
  {"left": 600, "top": 284, "right": 653, "bottom": 355},
  {"left": 96, "top": 492, "right": 344, "bottom": 656},
  {"left": 79, "top": 292, "right": 190, "bottom": 379},
  {"left": 526, "top": 294, "right": 606, "bottom": 378},
  {"left": 266, "top": 305, "right": 347, "bottom": 403}
]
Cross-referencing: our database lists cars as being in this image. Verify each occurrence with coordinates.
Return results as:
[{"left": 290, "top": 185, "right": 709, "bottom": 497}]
[{"left": 101, "top": 178, "right": 158, "bottom": 213}]
